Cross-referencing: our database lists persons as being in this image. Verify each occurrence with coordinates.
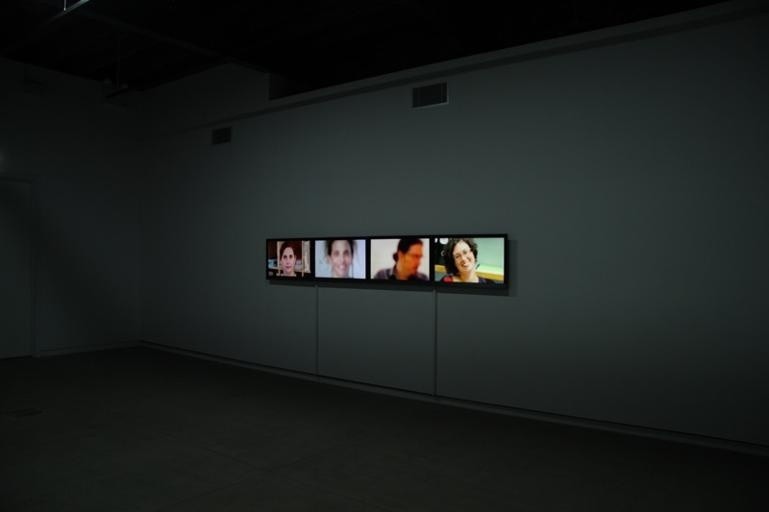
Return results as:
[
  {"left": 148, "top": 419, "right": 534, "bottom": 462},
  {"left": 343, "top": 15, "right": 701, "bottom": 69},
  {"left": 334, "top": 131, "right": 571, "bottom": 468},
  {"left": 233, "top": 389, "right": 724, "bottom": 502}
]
[
  {"left": 276, "top": 243, "right": 298, "bottom": 277},
  {"left": 372, "top": 238, "right": 429, "bottom": 281},
  {"left": 440, "top": 238, "right": 497, "bottom": 284},
  {"left": 322, "top": 240, "right": 357, "bottom": 278}
]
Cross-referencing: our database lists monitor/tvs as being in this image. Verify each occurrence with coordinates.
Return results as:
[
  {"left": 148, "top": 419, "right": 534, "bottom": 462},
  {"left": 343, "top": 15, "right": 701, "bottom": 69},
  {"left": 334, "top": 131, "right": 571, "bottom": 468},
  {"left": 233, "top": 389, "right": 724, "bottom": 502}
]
[{"left": 266, "top": 233, "right": 509, "bottom": 298}]
